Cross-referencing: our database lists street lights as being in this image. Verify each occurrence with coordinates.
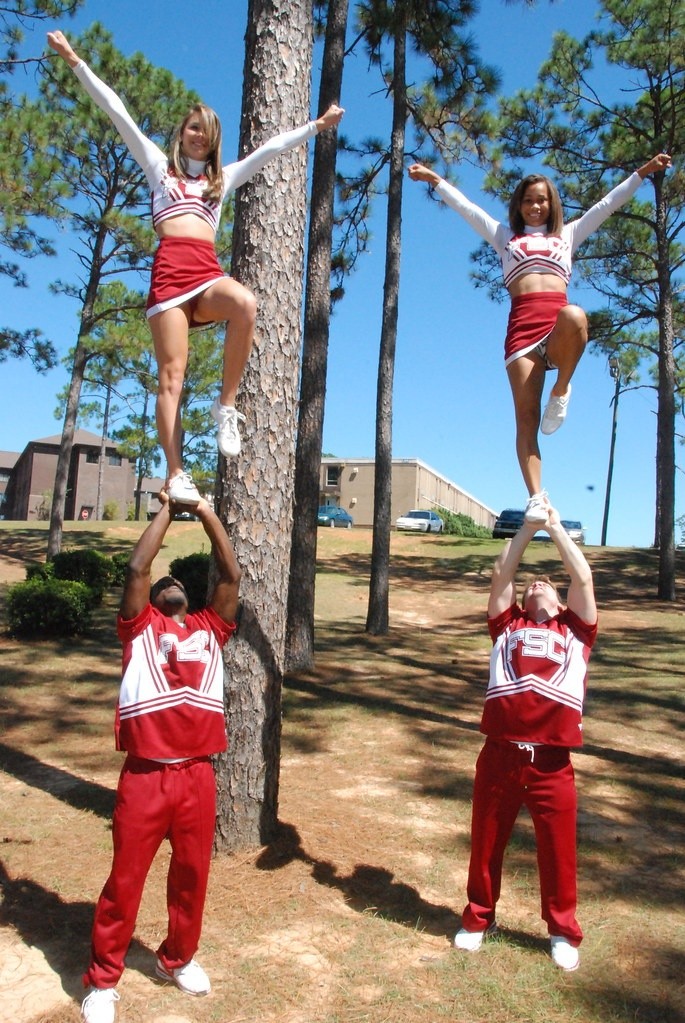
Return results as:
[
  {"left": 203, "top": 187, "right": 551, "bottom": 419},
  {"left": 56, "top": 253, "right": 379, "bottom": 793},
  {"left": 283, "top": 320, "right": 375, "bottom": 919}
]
[{"left": 601, "top": 357, "right": 638, "bottom": 546}]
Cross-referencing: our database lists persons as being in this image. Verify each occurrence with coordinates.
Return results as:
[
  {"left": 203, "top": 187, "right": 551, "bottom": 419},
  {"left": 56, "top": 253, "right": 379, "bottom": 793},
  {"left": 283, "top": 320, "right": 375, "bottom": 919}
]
[
  {"left": 455, "top": 498, "right": 599, "bottom": 972},
  {"left": 405, "top": 152, "right": 672, "bottom": 522},
  {"left": 81, "top": 489, "right": 241, "bottom": 1022},
  {"left": 41, "top": 30, "right": 346, "bottom": 505}
]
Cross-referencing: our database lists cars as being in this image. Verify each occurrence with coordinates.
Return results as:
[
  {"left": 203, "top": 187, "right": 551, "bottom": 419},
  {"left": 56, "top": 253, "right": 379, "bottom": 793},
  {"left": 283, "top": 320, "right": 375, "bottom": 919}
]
[
  {"left": 396, "top": 509, "right": 444, "bottom": 535},
  {"left": 532, "top": 530, "right": 553, "bottom": 542},
  {"left": 560, "top": 520, "right": 587, "bottom": 545},
  {"left": 317, "top": 505, "right": 354, "bottom": 529},
  {"left": 173, "top": 502, "right": 214, "bottom": 522}
]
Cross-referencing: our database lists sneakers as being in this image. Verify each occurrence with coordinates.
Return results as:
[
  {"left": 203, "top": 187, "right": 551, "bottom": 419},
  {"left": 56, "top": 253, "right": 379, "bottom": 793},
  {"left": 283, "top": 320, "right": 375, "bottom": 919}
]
[
  {"left": 549, "top": 934, "right": 580, "bottom": 972},
  {"left": 80, "top": 985, "right": 119, "bottom": 1023},
  {"left": 154, "top": 959, "right": 211, "bottom": 997},
  {"left": 166, "top": 472, "right": 201, "bottom": 505},
  {"left": 542, "top": 382, "right": 571, "bottom": 434},
  {"left": 209, "top": 395, "right": 247, "bottom": 457},
  {"left": 524, "top": 488, "right": 548, "bottom": 524},
  {"left": 454, "top": 927, "right": 484, "bottom": 953}
]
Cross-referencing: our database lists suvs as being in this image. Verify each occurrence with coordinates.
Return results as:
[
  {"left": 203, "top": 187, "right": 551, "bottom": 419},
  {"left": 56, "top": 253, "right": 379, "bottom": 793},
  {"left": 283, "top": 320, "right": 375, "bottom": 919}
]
[{"left": 493, "top": 508, "right": 525, "bottom": 539}]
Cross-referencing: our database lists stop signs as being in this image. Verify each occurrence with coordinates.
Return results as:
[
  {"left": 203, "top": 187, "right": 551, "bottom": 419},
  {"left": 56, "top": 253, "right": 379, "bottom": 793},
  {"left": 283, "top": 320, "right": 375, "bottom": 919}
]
[{"left": 82, "top": 510, "right": 88, "bottom": 519}]
